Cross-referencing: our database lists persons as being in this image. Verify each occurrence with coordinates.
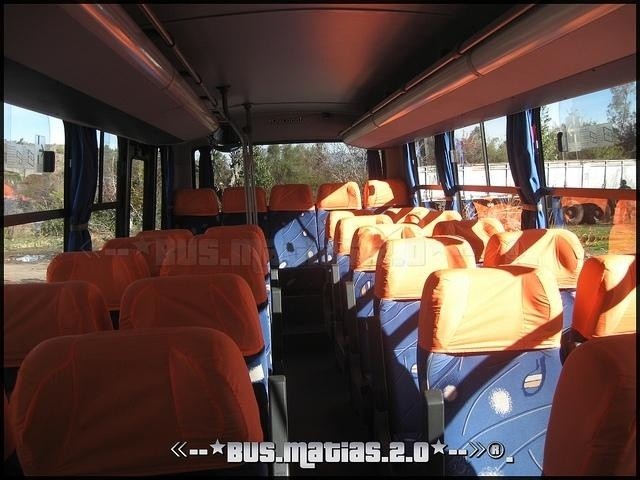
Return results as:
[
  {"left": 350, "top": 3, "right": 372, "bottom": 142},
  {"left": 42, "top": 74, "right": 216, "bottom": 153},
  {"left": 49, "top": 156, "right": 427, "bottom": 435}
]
[{"left": 618, "top": 179, "right": 631, "bottom": 189}]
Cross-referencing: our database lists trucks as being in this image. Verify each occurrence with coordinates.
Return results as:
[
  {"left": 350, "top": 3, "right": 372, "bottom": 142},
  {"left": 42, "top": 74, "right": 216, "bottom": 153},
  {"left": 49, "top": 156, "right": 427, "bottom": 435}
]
[{"left": 418, "top": 158, "right": 637, "bottom": 226}]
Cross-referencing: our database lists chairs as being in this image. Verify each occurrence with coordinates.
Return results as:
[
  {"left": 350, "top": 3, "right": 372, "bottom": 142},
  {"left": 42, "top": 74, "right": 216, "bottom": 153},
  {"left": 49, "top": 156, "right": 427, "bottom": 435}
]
[
  {"left": 268, "top": 182, "right": 318, "bottom": 274},
  {"left": 2, "top": 185, "right": 288, "bottom": 477},
  {"left": 317, "top": 178, "right": 636, "bottom": 477}
]
